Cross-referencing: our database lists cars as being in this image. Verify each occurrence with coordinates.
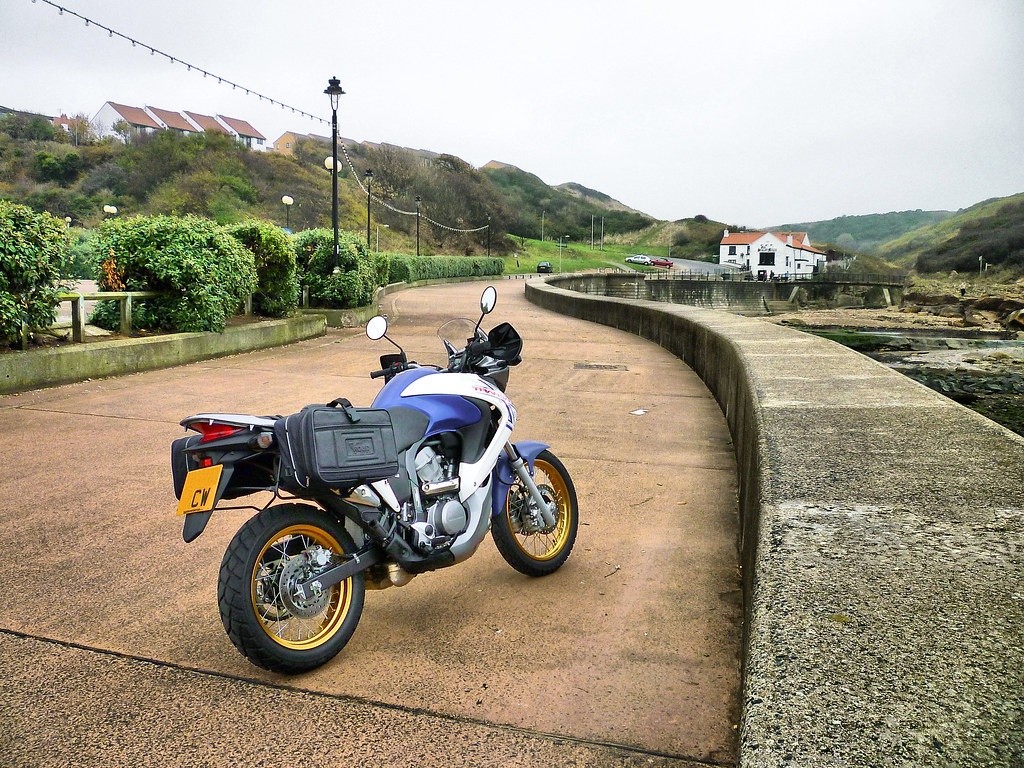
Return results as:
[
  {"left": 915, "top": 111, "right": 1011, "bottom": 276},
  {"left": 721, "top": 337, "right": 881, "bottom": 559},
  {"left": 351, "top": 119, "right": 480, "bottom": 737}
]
[
  {"left": 649, "top": 258, "right": 673, "bottom": 269},
  {"left": 537, "top": 261, "right": 553, "bottom": 273},
  {"left": 625, "top": 254, "right": 651, "bottom": 266}
]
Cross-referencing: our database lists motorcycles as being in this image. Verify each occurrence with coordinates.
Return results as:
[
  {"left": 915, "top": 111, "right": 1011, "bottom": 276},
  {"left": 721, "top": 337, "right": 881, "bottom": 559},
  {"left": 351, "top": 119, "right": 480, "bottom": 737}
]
[{"left": 165, "top": 287, "right": 580, "bottom": 678}]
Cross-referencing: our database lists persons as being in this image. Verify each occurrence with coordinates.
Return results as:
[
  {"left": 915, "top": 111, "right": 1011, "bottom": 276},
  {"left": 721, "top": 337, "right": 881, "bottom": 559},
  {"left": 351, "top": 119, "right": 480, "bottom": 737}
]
[{"left": 769, "top": 270, "right": 774, "bottom": 279}]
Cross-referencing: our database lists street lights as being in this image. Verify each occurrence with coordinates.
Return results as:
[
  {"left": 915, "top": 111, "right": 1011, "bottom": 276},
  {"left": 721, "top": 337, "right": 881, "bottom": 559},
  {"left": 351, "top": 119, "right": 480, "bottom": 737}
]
[
  {"left": 324, "top": 156, "right": 342, "bottom": 229},
  {"left": 559, "top": 235, "right": 570, "bottom": 274},
  {"left": 413, "top": 194, "right": 423, "bottom": 257},
  {"left": 282, "top": 194, "right": 294, "bottom": 229},
  {"left": 487, "top": 212, "right": 492, "bottom": 256},
  {"left": 364, "top": 167, "right": 374, "bottom": 250},
  {"left": 324, "top": 74, "right": 346, "bottom": 275}
]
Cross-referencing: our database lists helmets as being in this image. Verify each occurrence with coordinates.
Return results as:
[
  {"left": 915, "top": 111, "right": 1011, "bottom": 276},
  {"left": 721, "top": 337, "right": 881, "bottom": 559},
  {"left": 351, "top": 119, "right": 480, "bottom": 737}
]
[{"left": 473, "top": 355, "right": 510, "bottom": 393}]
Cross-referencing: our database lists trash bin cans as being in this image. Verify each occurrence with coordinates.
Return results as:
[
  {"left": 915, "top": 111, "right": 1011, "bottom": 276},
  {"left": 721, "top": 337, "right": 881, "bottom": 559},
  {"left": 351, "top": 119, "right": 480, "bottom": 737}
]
[{"left": 758, "top": 270, "right": 767, "bottom": 281}]
